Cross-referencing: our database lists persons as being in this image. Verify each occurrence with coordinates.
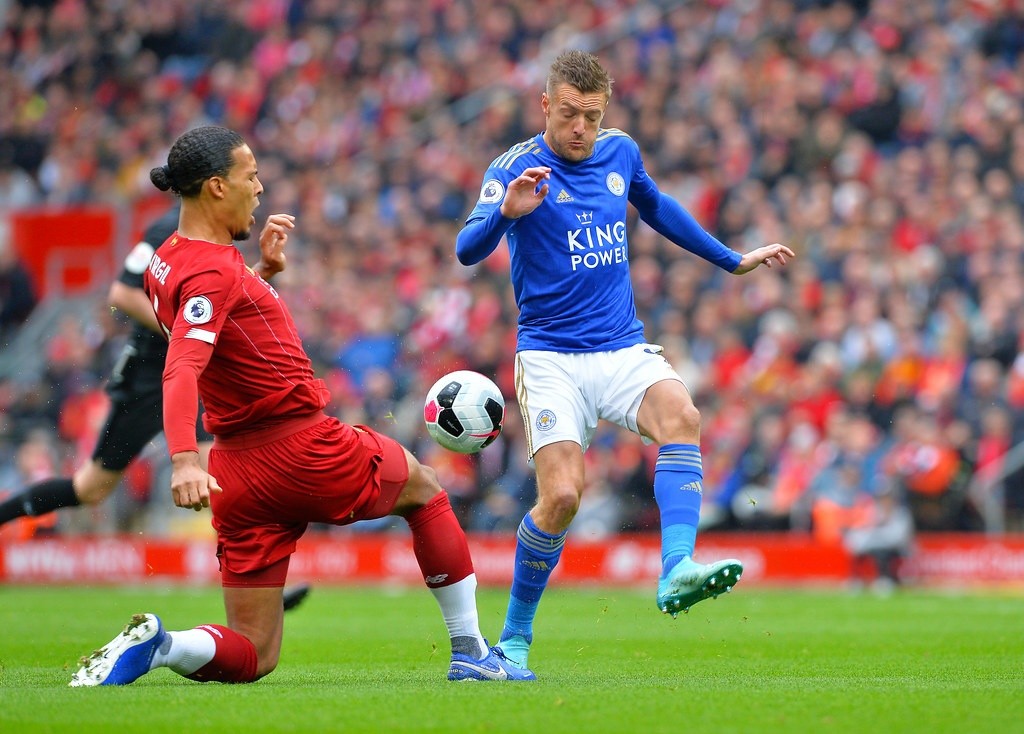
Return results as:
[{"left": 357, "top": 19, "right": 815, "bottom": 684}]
[
  {"left": 455, "top": 47, "right": 798, "bottom": 679},
  {"left": 2, "top": 0, "right": 1023, "bottom": 598},
  {"left": 0, "top": 197, "right": 314, "bottom": 615},
  {"left": 69, "top": 124, "right": 534, "bottom": 686}
]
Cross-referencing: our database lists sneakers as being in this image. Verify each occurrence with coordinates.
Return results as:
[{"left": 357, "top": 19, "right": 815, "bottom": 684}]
[
  {"left": 446, "top": 639, "right": 538, "bottom": 682},
  {"left": 492, "top": 636, "right": 530, "bottom": 671},
  {"left": 656, "top": 555, "right": 744, "bottom": 620},
  {"left": 66, "top": 613, "right": 166, "bottom": 686}
]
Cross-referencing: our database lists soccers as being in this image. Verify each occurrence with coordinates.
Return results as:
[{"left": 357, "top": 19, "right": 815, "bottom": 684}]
[{"left": 425, "top": 369, "right": 507, "bottom": 456}]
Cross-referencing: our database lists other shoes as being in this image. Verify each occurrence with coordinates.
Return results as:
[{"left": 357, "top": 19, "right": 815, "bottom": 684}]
[{"left": 282, "top": 582, "right": 308, "bottom": 612}]
